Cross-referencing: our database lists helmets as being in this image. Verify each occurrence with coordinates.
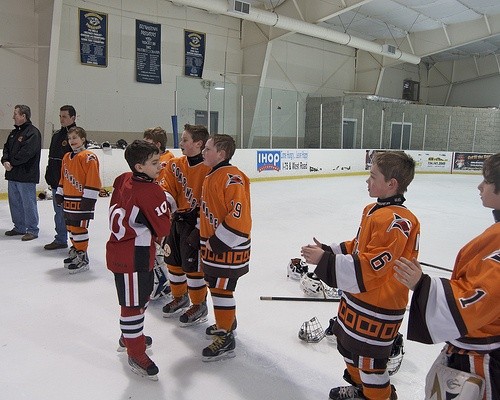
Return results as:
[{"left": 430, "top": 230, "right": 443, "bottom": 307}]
[
  {"left": 44, "top": 187, "right": 53, "bottom": 200},
  {"left": 299, "top": 272, "right": 323, "bottom": 297},
  {"left": 101, "top": 140, "right": 111, "bottom": 150},
  {"left": 287, "top": 258, "right": 308, "bottom": 280},
  {"left": 320, "top": 280, "right": 341, "bottom": 298},
  {"left": 116, "top": 139, "right": 127, "bottom": 149},
  {"left": 385, "top": 332, "right": 404, "bottom": 376},
  {"left": 324, "top": 318, "right": 337, "bottom": 343}
]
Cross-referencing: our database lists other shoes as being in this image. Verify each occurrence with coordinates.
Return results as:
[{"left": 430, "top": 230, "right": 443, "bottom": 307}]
[
  {"left": 158, "top": 284, "right": 171, "bottom": 298},
  {"left": 5, "top": 229, "right": 26, "bottom": 236},
  {"left": 44, "top": 242, "right": 68, "bottom": 250},
  {"left": 22, "top": 233, "right": 37, "bottom": 241}
]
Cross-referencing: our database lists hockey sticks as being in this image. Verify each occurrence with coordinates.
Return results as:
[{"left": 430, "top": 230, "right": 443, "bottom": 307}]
[
  {"left": 260, "top": 296, "right": 341, "bottom": 303},
  {"left": 415, "top": 261, "right": 453, "bottom": 273}
]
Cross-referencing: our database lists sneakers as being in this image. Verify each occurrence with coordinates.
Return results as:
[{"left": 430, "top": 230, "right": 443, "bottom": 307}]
[
  {"left": 117, "top": 333, "right": 152, "bottom": 353},
  {"left": 162, "top": 285, "right": 190, "bottom": 318},
  {"left": 201, "top": 329, "right": 236, "bottom": 362},
  {"left": 127, "top": 356, "right": 159, "bottom": 381},
  {"left": 67, "top": 250, "right": 89, "bottom": 274},
  {"left": 63, "top": 246, "right": 78, "bottom": 268},
  {"left": 205, "top": 318, "right": 237, "bottom": 340},
  {"left": 329, "top": 368, "right": 397, "bottom": 400},
  {"left": 179, "top": 292, "right": 208, "bottom": 327}
]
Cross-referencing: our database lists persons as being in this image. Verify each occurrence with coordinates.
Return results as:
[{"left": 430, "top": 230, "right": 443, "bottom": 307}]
[
  {"left": 392, "top": 152, "right": 500, "bottom": 400},
  {"left": 106, "top": 123, "right": 252, "bottom": 380},
  {"left": 45, "top": 105, "right": 81, "bottom": 251},
  {"left": 1, "top": 104, "right": 42, "bottom": 241},
  {"left": 55, "top": 127, "right": 101, "bottom": 275},
  {"left": 300, "top": 149, "right": 419, "bottom": 400}
]
